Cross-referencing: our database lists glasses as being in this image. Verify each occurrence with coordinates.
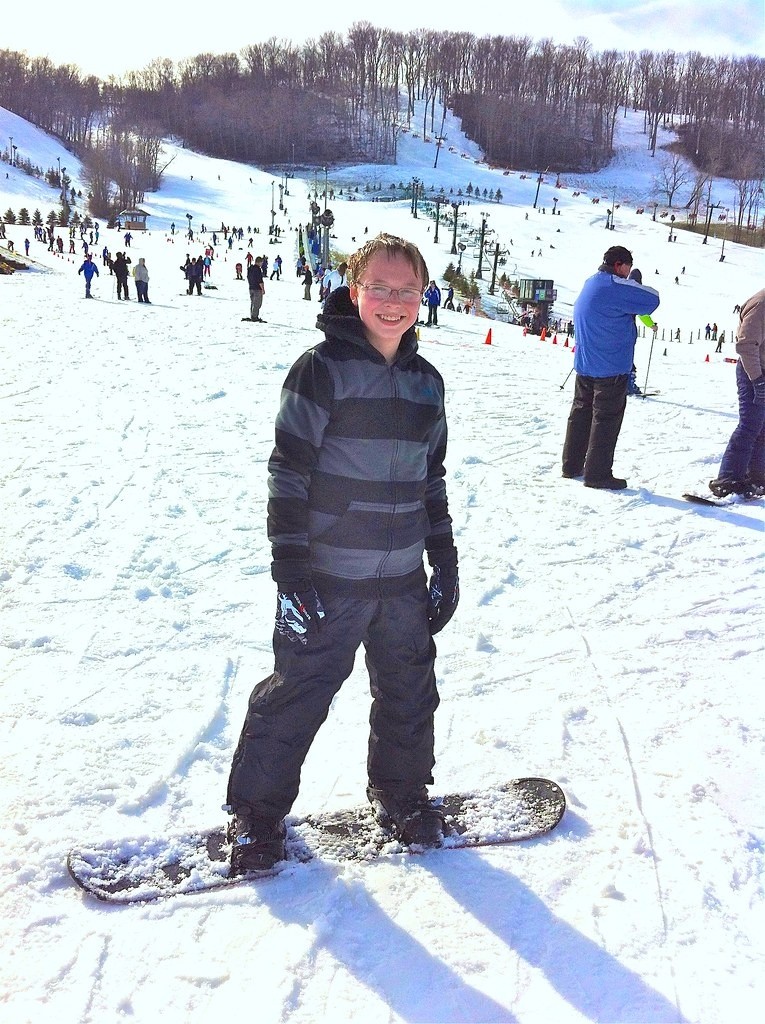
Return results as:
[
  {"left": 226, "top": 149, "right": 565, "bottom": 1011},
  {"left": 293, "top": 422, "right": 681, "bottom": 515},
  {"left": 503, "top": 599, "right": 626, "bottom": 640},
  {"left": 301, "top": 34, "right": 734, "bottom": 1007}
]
[{"left": 357, "top": 281, "right": 424, "bottom": 303}]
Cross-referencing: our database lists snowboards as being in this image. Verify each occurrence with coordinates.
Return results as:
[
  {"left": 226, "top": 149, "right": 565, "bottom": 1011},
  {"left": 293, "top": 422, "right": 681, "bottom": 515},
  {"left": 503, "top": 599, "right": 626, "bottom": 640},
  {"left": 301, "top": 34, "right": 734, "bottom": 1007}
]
[
  {"left": 67, "top": 775, "right": 566, "bottom": 903},
  {"left": 241, "top": 318, "right": 267, "bottom": 323},
  {"left": 682, "top": 490, "right": 762, "bottom": 506},
  {"left": 180, "top": 266, "right": 188, "bottom": 273}
]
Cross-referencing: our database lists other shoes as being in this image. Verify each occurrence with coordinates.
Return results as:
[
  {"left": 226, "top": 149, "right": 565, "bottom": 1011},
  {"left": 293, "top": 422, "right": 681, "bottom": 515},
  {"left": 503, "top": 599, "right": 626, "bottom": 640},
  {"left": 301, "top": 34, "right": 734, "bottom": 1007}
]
[
  {"left": 251, "top": 316, "right": 260, "bottom": 321},
  {"left": 562, "top": 467, "right": 584, "bottom": 478},
  {"left": 584, "top": 477, "right": 627, "bottom": 490}
]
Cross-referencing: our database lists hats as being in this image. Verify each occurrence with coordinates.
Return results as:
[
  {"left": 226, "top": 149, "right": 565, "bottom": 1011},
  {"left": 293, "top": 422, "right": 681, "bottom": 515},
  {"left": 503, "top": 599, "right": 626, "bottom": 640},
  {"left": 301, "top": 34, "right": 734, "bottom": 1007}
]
[
  {"left": 304, "top": 265, "right": 309, "bottom": 268},
  {"left": 629, "top": 269, "right": 642, "bottom": 285}
]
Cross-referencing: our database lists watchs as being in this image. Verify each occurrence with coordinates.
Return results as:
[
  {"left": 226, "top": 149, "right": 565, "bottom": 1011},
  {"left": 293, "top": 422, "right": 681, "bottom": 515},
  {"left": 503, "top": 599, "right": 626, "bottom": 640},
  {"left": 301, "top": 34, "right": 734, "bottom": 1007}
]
[{"left": 627, "top": 269, "right": 658, "bottom": 396}]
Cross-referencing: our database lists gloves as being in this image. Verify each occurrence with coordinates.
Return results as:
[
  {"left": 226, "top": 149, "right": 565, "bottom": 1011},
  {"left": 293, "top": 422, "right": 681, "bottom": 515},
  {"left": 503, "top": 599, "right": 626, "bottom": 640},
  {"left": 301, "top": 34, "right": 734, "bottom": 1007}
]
[
  {"left": 752, "top": 374, "right": 765, "bottom": 407},
  {"left": 272, "top": 580, "right": 325, "bottom": 650},
  {"left": 426, "top": 548, "right": 460, "bottom": 635}
]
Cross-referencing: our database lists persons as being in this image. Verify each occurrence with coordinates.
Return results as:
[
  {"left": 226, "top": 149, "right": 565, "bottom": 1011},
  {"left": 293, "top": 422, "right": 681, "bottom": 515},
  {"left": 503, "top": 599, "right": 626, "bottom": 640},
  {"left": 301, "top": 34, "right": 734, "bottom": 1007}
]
[
  {"left": 0, "top": 154, "right": 133, "bottom": 300},
  {"left": 171, "top": 80, "right": 765, "bottom": 356},
  {"left": 561, "top": 246, "right": 660, "bottom": 491},
  {"left": 135, "top": 257, "right": 151, "bottom": 303},
  {"left": 247, "top": 257, "right": 265, "bottom": 321},
  {"left": 709, "top": 288, "right": 765, "bottom": 499},
  {"left": 180, "top": 257, "right": 203, "bottom": 295},
  {"left": 78, "top": 254, "right": 99, "bottom": 298},
  {"left": 224, "top": 233, "right": 459, "bottom": 878},
  {"left": 302, "top": 265, "right": 312, "bottom": 300}
]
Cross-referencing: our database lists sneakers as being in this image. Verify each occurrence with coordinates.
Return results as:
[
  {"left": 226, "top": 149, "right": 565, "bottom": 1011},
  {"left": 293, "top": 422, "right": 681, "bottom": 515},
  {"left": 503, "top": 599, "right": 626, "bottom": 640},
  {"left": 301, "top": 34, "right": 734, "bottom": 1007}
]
[
  {"left": 367, "top": 784, "right": 444, "bottom": 843},
  {"left": 628, "top": 385, "right": 640, "bottom": 394},
  {"left": 711, "top": 471, "right": 765, "bottom": 499},
  {"left": 235, "top": 819, "right": 285, "bottom": 869}
]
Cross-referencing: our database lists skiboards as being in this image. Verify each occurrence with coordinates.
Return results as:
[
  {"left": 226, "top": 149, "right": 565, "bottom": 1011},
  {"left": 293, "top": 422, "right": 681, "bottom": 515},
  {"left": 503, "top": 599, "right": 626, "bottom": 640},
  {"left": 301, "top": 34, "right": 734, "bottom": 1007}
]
[
  {"left": 630, "top": 390, "right": 661, "bottom": 396},
  {"left": 81, "top": 295, "right": 101, "bottom": 299}
]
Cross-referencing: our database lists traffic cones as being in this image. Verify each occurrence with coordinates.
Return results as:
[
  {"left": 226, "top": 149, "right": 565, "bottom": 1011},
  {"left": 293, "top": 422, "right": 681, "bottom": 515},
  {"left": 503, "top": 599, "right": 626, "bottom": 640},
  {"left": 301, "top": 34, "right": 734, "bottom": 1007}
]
[
  {"left": 571, "top": 346, "right": 575, "bottom": 352},
  {"left": 564, "top": 337, "right": 569, "bottom": 347},
  {"left": 482, "top": 328, "right": 492, "bottom": 344},
  {"left": 540, "top": 328, "right": 546, "bottom": 341},
  {"left": 705, "top": 354, "right": 710, "bottom": 362},
  {"left": 523, "top": 327, "right": 527, "bottom": 337},
  {"left": 663, "top": 349, "right": 667, "bottom": 356},
  {"left": 416, "top": 328, "right": 421, "bottom": 341},
  {"left": 553, "top": 335, "right": 557, "bottom": 344}
]
